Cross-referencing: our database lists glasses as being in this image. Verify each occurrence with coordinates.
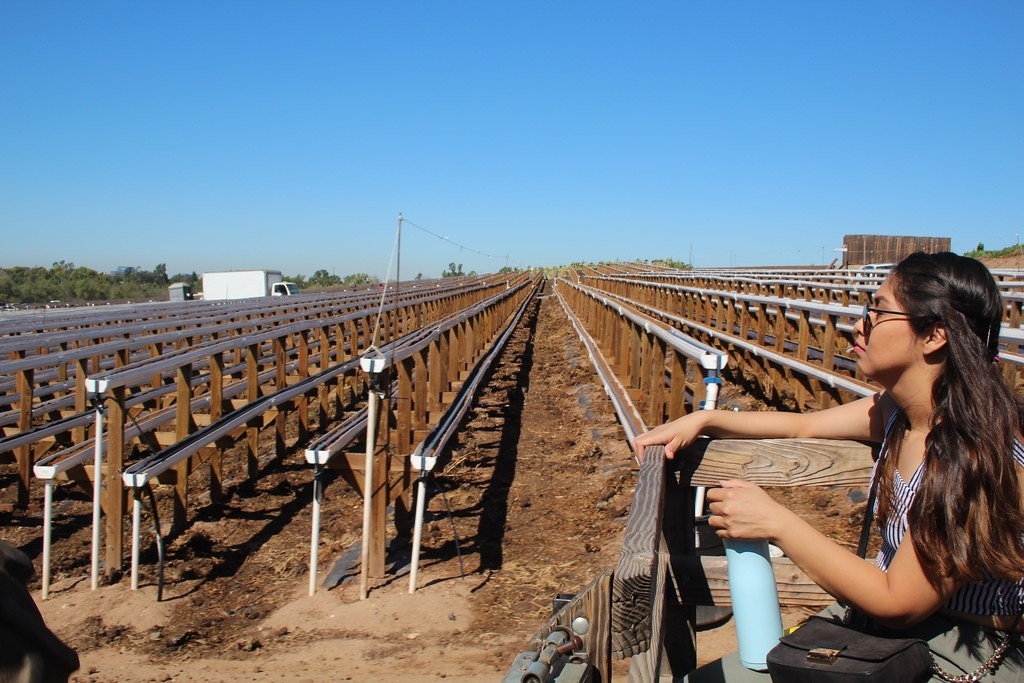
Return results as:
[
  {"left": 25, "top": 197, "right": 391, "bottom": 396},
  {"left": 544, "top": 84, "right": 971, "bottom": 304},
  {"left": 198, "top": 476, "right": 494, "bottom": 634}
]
[{"left": 862, "top": 301, "right": 917, "bottom": 336}]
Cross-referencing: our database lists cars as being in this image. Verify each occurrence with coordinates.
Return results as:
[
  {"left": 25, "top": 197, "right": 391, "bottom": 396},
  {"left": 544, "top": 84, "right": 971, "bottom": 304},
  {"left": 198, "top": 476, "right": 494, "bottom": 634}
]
[{"left": 854, "top": 263, "right": 898, "bottom": 277}]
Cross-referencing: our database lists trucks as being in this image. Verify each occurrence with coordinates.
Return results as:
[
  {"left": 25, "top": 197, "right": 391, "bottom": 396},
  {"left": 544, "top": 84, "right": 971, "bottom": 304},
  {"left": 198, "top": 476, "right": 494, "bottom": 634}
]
[{"left": 202, "top": 269, "right": 301, "bottom": 305}]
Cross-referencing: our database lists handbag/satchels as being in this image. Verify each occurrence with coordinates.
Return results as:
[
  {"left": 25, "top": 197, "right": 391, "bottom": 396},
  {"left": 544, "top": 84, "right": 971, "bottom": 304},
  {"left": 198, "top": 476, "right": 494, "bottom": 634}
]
[{"left": 766, "top": 613, "right": 935, "bottom": 683}]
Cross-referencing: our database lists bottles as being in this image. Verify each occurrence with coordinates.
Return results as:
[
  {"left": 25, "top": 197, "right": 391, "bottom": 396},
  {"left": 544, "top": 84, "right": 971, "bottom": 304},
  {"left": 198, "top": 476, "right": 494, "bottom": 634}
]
[{"left": 720, "top": 536, "right": 786, "bottom": 673}]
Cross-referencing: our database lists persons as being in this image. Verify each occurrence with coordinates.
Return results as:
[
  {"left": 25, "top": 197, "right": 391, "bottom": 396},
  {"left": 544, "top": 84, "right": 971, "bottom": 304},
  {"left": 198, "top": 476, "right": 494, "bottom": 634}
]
[
  {"left": 632, "top": 251, "right": 1024, "bottom": 683},
  {"left": 0, "top": 540, "right": 80, "bottom": 683}
]
